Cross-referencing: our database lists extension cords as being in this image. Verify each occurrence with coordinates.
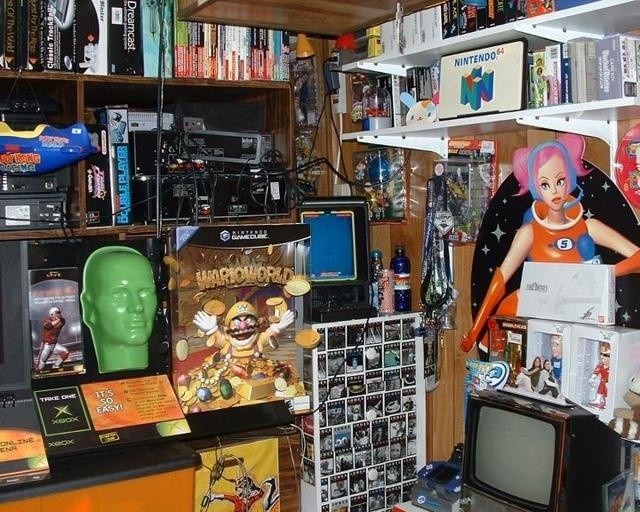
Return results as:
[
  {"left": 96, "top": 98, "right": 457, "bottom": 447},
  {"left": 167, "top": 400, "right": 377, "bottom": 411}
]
[{"left": 329, "top": 73, "right": 346, "bottom": 115}]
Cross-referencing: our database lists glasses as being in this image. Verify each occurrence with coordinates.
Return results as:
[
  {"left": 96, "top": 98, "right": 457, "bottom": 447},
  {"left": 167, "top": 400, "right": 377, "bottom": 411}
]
[{"left": 601, "top": 352, "right": 609, "bottom": 357}]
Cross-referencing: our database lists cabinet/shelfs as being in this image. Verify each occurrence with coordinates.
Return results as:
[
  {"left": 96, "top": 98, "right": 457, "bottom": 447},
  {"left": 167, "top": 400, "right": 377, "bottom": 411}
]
[
  {"left": 1, "top": 69, "right": 298, "bottom": 241},
  {"left": 339, "top": 1, "right": 640, "bottom": 79},
  {"left": 340, "top": 96, "right": 640, "bottom": 182}
]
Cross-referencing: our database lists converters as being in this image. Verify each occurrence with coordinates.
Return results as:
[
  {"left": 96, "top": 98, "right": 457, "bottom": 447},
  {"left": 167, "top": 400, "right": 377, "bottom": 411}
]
[{"left": 326, "top": 72, "right": 340, "bottom": 90}]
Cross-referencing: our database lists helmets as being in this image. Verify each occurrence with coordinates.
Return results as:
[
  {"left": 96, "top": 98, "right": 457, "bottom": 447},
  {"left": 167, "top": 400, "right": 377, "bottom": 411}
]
[
  {"left": 236, "top": 477, "right": 252, "bottom": 489},
  {"left": 49, "top": 307, "right": 60, "bottom": 316}
]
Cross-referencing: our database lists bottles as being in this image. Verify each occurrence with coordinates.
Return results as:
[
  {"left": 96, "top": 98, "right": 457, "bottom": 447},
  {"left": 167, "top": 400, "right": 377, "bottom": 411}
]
[
  {"left": 390, "top": 247, "right": 411, "bottom": 312},
  {"left": 378, "top": 269, "right": 394, "bottom": 313},
  {"left": 369, "top": 250, "right": 383, "bottom": 311}
]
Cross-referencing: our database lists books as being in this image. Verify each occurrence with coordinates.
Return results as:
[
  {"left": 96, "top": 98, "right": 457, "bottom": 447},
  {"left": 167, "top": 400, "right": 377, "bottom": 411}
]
[
  {"left": 174, "top": 21, "right": 290, "bottom": 82},
  {"left": 602, "top": 469, "right": 635, "bottom": 512},
  {"left": 532, "top": 37, "right": 595, "bottom": 107}
]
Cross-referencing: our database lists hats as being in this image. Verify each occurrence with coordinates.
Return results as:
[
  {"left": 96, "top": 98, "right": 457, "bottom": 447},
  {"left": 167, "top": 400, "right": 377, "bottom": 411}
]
[{"left": 225, "top": 301, "right": 258, "bottom": 327}]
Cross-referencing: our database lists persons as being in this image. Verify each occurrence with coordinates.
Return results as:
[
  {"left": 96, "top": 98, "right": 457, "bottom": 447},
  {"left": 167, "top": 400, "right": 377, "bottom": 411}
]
[
  {"left": 510, "top": 357, "right": 541, "bottom": 393},
  {"left": 34, "top": 307, "right": 70, "bottom": 375},
  {"left": 461, "top": 132, "right": 640, "bottom": 353},
  {"left": 79, "top": 246, "right": 157, "bottom": 373},
  {"left": 549, "top": 334, "right": 562, "bottom": 382},
  {"left": 538, "top": 360, "right": 560, "bottom": 400},
  {"left": 589, "top": 343, "right": 611, "bottom": 409},
  {"left": 193, "top": 301, "right": 294, "bottom": 362}
]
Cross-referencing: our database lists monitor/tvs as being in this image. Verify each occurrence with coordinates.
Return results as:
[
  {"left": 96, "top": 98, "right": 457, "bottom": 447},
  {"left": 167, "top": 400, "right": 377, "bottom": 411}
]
[
  {"left": 300, "top": 210, "right": 361, "bottom": 282},
  {"left": 461, "top": 390, "right": 599, "bottom": 512}
]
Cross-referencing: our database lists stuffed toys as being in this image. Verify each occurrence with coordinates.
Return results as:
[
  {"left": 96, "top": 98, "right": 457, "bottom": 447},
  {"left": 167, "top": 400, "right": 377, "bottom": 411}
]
[{"left": 608, "top": 373, "right": 640, "bottom": 440}]
